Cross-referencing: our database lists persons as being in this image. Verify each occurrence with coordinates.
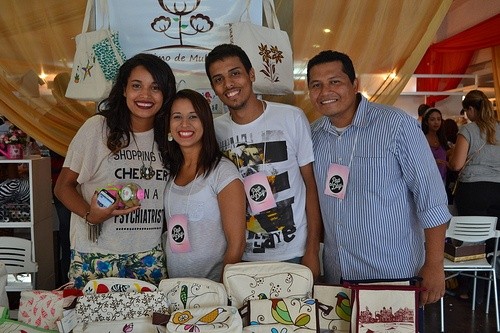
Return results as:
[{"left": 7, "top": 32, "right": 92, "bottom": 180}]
[
  {"left": 422, "top": 108, "right": 447, "bottom": 188},
  {"left": 164, "top": 89, "right": 246, "bottom": 283},
  {"left": 444, "top": 90, "right": 500, "bottom": 303},
  {"left": 418, "top": 104, "right": 430, "bottom": 121},
  {"left": 54, "top": 53, "right": 176, "bottom": 288},
  {"left": 205, "top": 44, "right": 321, "bottom": 284},
  {"left": 307, "top": 50, "right": 452, "bottom": 333},
  {"left": 40, "top": 72, "right": 89, "bottom": 249}
]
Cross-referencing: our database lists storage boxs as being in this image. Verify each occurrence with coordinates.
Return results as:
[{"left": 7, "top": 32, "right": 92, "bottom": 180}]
[{"left": 444, "top": 240, "right": 486, "bottom": 262}]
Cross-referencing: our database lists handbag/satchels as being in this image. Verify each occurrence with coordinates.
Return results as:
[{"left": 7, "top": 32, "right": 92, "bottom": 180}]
[
  {"left": 225, "top": 0, "right": 294, "bottom": 97},
  {"left": 0, "top": 260, "right": 429, "bottom": 333},
  {"left": 65, "top": 0, "right": 126, "bottom": 101}
]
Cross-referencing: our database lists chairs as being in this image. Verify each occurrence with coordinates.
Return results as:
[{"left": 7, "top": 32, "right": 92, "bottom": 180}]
[
  {"left": 0, "top": 237, "right": 39, "bottom": 319},
  {"left": 423, "top": 216, "right": 500, "bottom": 332}
]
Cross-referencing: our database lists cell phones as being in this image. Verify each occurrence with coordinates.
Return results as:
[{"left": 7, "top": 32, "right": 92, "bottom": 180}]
[{"left": 96, "top": 188, "right": 117, "bottom": 209}]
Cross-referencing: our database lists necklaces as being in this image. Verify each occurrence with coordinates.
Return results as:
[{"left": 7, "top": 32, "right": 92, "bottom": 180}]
[{"left": 130, "top": 129, "right": 154, "bottom": 180}]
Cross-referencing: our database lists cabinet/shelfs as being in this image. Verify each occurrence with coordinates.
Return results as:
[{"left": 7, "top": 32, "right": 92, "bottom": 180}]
[{"left": 0, "top": 155, "right": 55, "bottom": 291}]
